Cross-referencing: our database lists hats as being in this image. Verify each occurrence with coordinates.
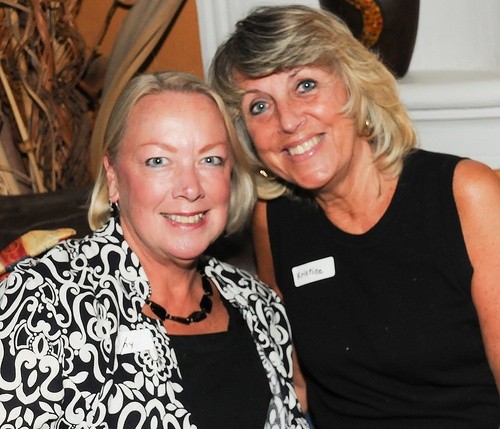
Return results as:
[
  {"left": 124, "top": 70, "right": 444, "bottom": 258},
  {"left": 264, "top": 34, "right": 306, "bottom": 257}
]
[{"left": 319, "top": 0, "right": 420, "bottom": 80}]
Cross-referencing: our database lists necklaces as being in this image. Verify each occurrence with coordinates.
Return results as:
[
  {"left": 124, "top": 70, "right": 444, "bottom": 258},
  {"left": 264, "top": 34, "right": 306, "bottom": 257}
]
[{"left": 145, "top": 265, "right": 213, "bottom": 325}]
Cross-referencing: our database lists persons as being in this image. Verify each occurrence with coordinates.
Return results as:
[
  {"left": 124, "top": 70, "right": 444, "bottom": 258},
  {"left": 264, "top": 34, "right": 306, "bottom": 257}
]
[
  {"left": 0, "top": 72, "right": 310, "bottom": 429},
  {"left": 207, "top": 6, "right": 500, "bottom": 429}
]
